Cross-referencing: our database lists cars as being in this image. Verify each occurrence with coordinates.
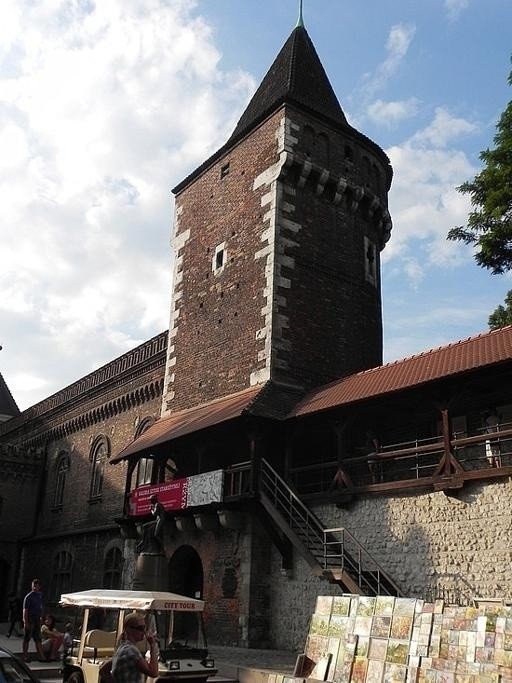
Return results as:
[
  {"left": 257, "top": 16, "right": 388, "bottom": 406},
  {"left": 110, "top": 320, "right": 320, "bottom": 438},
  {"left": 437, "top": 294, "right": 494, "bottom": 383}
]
[{"left": 0, "top": 645, "right": 42, "bottom": 683}]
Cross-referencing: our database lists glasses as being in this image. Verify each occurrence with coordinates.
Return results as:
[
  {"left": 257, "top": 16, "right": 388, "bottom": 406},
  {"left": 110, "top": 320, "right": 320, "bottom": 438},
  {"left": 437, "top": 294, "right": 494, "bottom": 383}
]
[{"left": 128, "top": 623, "right": 146, "bottom": 631}]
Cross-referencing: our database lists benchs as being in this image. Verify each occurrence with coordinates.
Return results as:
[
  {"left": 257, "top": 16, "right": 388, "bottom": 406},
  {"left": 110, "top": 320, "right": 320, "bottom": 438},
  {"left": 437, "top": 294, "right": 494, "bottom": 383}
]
[{"left": 66, "top": 624, "right": 150, "bottom": 665}]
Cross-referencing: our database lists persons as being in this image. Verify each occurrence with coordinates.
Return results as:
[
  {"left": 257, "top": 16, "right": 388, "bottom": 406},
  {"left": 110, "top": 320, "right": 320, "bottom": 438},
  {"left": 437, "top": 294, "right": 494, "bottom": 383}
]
[
  {"left": 22, "top": 578, "right": 50, "bottom": 663},
  {"left": 149, "top": 493, "right": 169, "bottom": 556},
  {"left": 80, "top": 606, "right": 111, "bottom": 631},
  {"left": 63, "top": 620, "right": 76, "bottom": 657},
  {"left": 365, "top": 428, "right": 383, "bottom": 483},
  {"left": 142, "top": 608, "right": 159, "bottom": 636},
  {"left": 41, "top": 613, "right": 66, "bottom": 661},
  {"left": 6, "top": 590, "right": 24, "bottom": 637},
  {"left": 111, "top": 613, "right": 159, "bottom": 682}
]
